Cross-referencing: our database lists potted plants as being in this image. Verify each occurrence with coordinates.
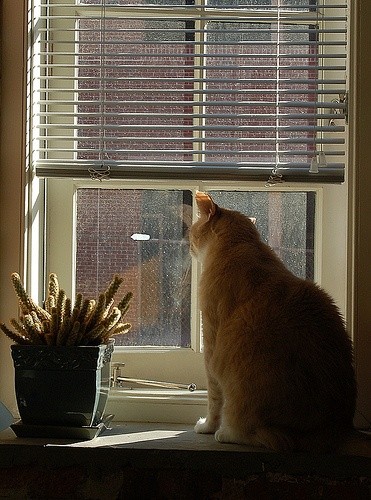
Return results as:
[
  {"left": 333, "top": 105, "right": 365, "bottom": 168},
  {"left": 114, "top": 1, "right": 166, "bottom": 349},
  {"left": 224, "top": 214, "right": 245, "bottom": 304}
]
[{"left": 0, "top": 272, "right": 133, "bottom": 439}]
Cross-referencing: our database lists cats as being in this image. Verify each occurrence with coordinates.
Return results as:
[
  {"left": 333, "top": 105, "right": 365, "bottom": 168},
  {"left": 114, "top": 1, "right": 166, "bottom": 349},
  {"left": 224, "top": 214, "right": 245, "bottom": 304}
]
[{"left": 188, "top": 190, "right": 359, "bottom": 453}]
